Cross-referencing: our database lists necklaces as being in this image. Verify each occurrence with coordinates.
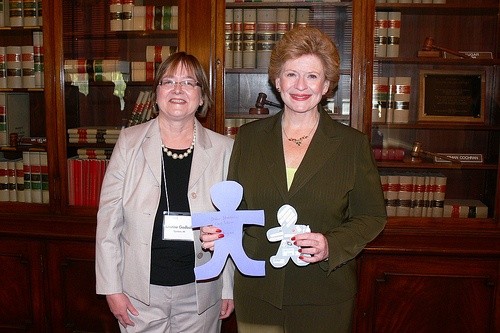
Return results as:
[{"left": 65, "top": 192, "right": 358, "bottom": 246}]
[
  {"left": 161, "top": 122, "right": 197, "bottom": 159},
  {"left": 282, "top": 115, "right": 319, "bottom": 146}
]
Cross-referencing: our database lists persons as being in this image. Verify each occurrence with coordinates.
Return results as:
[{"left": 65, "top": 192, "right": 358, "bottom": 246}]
[
  {"left": 199, "top": 27, "right": 387, "bottom": 333},
  {"left": 95, "top": 52, "right": 235, "bottom": 333}
]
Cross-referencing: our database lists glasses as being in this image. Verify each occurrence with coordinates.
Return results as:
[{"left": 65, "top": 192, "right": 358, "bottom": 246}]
[{"left": 155, "top": 77, "right": 202, "bottom": 89}]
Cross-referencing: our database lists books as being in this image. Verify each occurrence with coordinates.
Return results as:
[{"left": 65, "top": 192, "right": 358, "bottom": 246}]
[
  {"left": 380, "top": 172, "right": 488, "bottom": 219},
  {"left": 62, "top": 0, "right": 178, "bottom": 82},
  {"left": 371, "top": 76, "right": 411, "bottom": 123},
  {"left": 376, "top": 12, "right": 388, "bottom": 57},
  {"left": 387, "top": 12, "right": 402, "bottom": 58},
  {"left": 225, "top": 0, "right": 353, "bottom": 71},
  {"left": 67, "top": 86, "right": 160, "bottom": 207},
  {"left": 0, "top": 91, "right": 50, "bottom": 204},
  {"left": 0, "top": 0, "right": 44, "bottom": 89}
]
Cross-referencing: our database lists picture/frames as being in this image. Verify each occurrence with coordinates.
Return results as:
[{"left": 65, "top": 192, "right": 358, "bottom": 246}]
[{"left": 417, "top": 69, "right": 486, "bottom": 123}]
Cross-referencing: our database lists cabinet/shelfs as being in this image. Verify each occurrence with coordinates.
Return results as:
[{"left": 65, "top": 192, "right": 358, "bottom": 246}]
[{"left": 0, "top": 0, "right": 500, "bottom": 237}]
